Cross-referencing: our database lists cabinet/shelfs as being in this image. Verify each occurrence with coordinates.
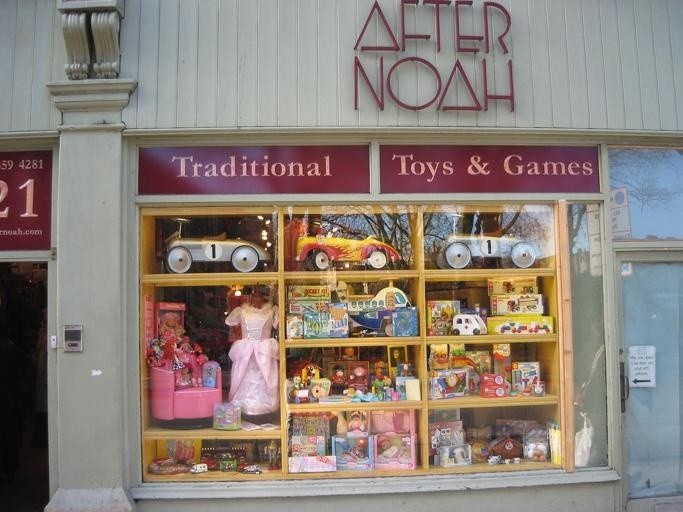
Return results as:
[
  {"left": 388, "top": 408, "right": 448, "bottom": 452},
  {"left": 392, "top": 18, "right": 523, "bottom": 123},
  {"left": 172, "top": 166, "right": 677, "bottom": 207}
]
[{"left": 140, "top": 200, "right": 573, "bottom": 481}]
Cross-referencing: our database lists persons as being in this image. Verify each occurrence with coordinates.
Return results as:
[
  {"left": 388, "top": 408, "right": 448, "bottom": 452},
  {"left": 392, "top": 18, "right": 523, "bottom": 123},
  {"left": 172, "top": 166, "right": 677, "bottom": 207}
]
[
  {"left": 343, "top": 347, "right": 357, "bottom": 360},
  {"left": 150, "top": 314, "right": 203, "bottom": 387},
  {"left": 377, "top": 435, "right": 406, "bottom": 458},
  {"left": 345, "top": 437, "right": 368, "bottom": 459},
  {"left": 348, "top": 410, "right": 363, "bottom": 433},
  {"left": 375, "top": 349, "right": 411, "bottom": 391},
  {"left": 350, "top": 367, "right": 367, "bottom": 391},
  {"left": 289, "top": 375, "right": 303, "bottom": 399},
  {"left": 225, "top": 293, "right": 280, "bottom": 415},
  {"left": 332, "top": 368, "right": 345, "bottom": 390}
]
